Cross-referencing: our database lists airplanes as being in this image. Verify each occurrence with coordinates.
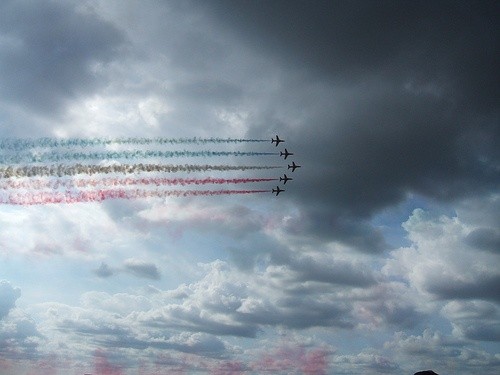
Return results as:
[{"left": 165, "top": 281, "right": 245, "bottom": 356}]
[
  {"left": 280, "top": 173, "right": 294, "bottom": 184},
  {"left": 279, "top": 148, "right": 294, "bottom": 159},
  {"left": 288, "top": 161, "right": 301, "bottom": 172},
  {"left": 272, "top": 186, "right": 285, "bottom": 197},
  {"left": 271, "top": 135, "right": 285, "bottom": 147}
]
